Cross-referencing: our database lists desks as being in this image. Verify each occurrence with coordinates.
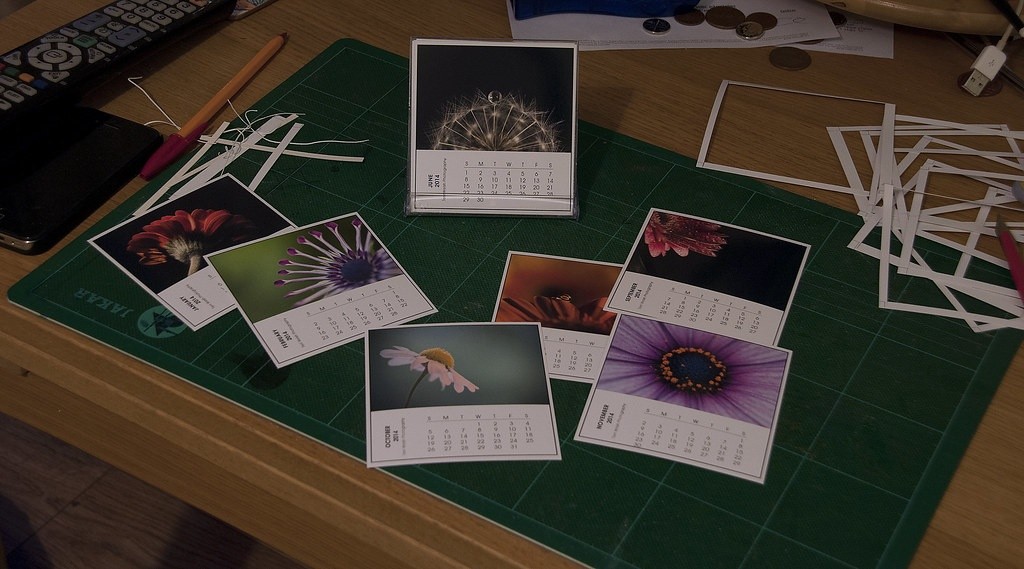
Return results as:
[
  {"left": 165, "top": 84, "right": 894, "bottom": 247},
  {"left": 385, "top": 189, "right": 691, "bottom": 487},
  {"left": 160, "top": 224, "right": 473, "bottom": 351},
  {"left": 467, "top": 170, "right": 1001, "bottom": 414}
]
[{"left": 0, "top": 0, "right": 1024, "bottom": 569}]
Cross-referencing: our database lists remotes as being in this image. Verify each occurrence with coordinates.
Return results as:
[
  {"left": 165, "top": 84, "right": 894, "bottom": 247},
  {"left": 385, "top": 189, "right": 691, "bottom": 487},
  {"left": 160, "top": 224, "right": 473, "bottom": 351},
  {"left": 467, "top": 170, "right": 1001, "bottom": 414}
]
[{"left": 0, "top": 0, "right": 239, "bottom": 131}]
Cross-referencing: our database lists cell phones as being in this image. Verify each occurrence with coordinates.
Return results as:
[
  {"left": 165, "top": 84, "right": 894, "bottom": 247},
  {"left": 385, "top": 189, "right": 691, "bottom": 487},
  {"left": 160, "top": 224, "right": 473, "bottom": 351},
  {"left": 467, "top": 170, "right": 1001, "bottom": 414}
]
[{"left": 0, "top": 106, "right": 162, "bottom": 251}]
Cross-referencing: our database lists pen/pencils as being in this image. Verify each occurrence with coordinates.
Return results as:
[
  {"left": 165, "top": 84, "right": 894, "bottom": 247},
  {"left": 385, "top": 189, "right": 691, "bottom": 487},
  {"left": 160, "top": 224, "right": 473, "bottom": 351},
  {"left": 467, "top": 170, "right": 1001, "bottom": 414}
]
[
  {"left": 992, "top": 0, "right": 1024, "bottom": 37},
  {"left": 138, "top": 30, "right": 289, "bottom": 182},
  {"left": 993, "top": 212, "right": 1024, "bottom": 302}
]
